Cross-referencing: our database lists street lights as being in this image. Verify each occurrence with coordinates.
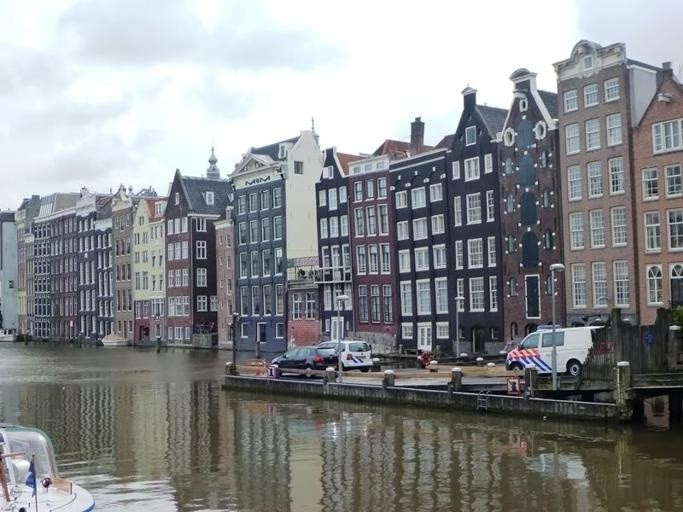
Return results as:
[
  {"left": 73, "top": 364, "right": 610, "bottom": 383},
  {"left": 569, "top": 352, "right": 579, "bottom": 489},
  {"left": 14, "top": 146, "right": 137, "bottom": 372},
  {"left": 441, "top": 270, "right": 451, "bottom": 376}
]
[
  {"left": 549, "top": 261, "right": 567, "bottom": 392},
  {"left": 454, "top": 295, "right": 465, "bottom": 363},
  {"left": 334, "top": 294, "right": 349, "bottom": 385}
]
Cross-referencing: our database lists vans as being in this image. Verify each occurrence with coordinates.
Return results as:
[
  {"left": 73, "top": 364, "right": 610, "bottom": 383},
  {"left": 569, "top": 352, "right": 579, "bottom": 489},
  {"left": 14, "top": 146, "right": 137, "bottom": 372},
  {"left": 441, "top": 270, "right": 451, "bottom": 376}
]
[
  {"left": 316, "top": 340, "right": 374, "bottom": 372},
  {"left": 504, "top": 324, "right": 612, "bottom": 376}
]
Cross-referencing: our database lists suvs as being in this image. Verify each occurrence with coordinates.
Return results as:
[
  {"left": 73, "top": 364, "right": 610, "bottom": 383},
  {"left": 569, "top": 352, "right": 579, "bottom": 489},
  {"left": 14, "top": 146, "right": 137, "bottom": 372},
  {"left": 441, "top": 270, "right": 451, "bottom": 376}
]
[{"left": 267, "top": 345, "right": 341, "bottom": 379}]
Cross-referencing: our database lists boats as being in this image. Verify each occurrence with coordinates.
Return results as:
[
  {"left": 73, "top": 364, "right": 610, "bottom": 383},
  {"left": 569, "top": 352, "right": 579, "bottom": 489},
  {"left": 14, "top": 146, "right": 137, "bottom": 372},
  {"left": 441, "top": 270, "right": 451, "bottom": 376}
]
[
  {"left": 0, "top": 333, "right": 19, "bottom": 342},
  {"left": 100, "top": 336, "right": 127, "bottom": 347},
  {"left": 0, "top": 423, "right": 96, "bottom": 512}
]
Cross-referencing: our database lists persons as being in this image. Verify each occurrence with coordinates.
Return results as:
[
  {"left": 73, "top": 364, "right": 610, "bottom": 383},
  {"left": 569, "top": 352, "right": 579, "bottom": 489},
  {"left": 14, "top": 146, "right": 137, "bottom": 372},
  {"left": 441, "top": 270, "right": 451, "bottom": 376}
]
[{"left": 0, "top": 434, "right": 10, "bottom": 501}]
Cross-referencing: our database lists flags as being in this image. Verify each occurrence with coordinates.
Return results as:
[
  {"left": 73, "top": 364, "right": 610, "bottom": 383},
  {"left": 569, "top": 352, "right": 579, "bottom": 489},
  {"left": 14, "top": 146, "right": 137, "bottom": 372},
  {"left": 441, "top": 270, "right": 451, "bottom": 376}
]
[{"left": 26, "top": 461, "right": 36, "bottom": 498}]
